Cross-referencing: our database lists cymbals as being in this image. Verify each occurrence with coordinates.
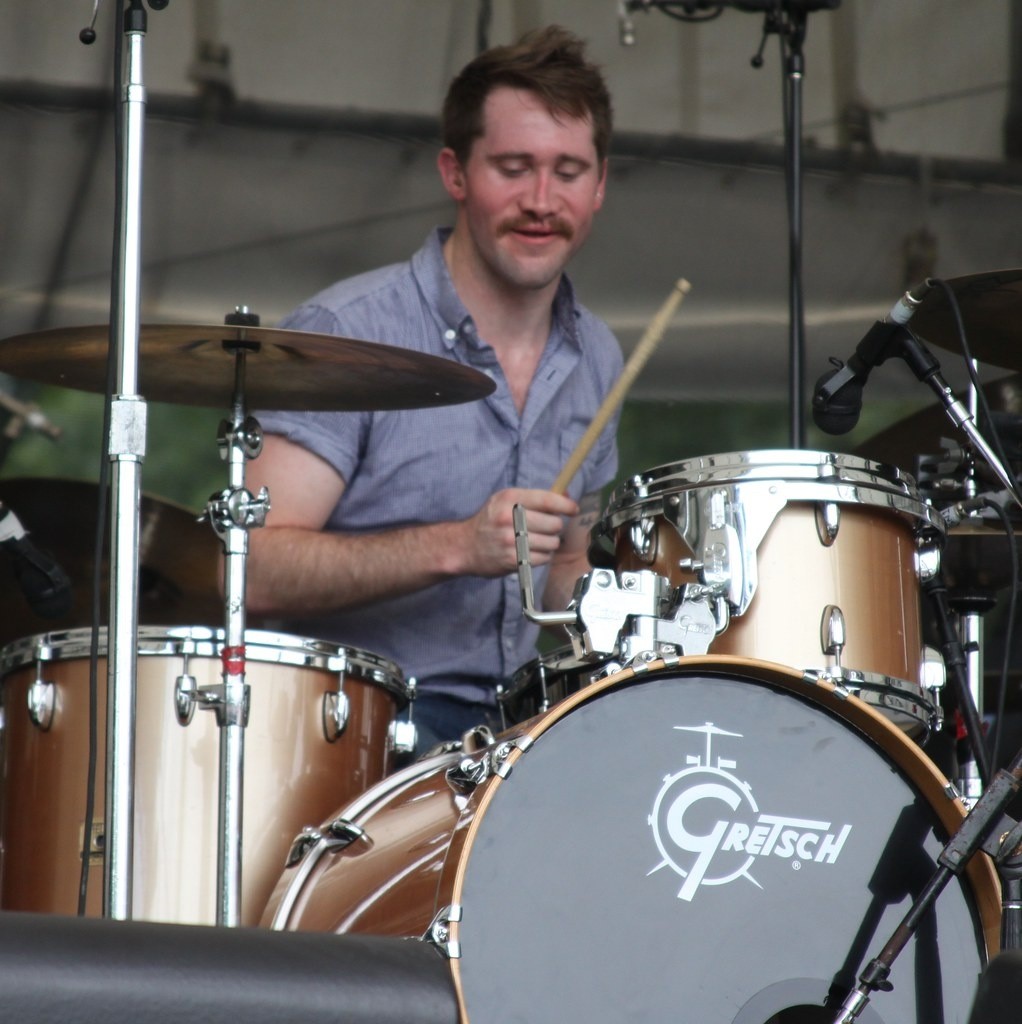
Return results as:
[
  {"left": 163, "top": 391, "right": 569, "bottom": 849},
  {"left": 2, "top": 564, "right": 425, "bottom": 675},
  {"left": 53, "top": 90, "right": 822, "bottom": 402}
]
[
  {"left": 908, "top": 266, "right": 1022, "bottom": 366},
  {"left": 0, "top": 320, "right": 500, "bottom": 415}
]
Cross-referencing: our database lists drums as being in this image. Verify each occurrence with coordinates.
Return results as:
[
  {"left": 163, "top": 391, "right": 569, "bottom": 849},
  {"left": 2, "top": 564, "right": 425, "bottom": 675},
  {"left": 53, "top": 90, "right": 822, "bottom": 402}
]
[
  {"left": 1, "top": 471, "right": 220, "bottom": 621},
  {"left": 1, "top": 621, "right": 415, "bottom": 930},
  {"left": 261, "top": 652, "right": 1006, "bottom": 1024},
  {"left": 604, "top": 447, "right": 950, "bottom": 732}
]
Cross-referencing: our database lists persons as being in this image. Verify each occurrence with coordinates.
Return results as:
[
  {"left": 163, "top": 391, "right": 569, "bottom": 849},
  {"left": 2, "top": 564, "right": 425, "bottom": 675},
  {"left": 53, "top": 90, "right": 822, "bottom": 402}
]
[{"left": 217, "top": 29, "right": 631, "bottom": 769}]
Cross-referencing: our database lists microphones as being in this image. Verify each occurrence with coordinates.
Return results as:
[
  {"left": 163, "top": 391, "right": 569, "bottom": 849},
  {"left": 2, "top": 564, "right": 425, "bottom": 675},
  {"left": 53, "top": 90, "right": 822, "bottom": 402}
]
[{"left": 812, "top": 278, "right": 936, "bottom": 434}]
